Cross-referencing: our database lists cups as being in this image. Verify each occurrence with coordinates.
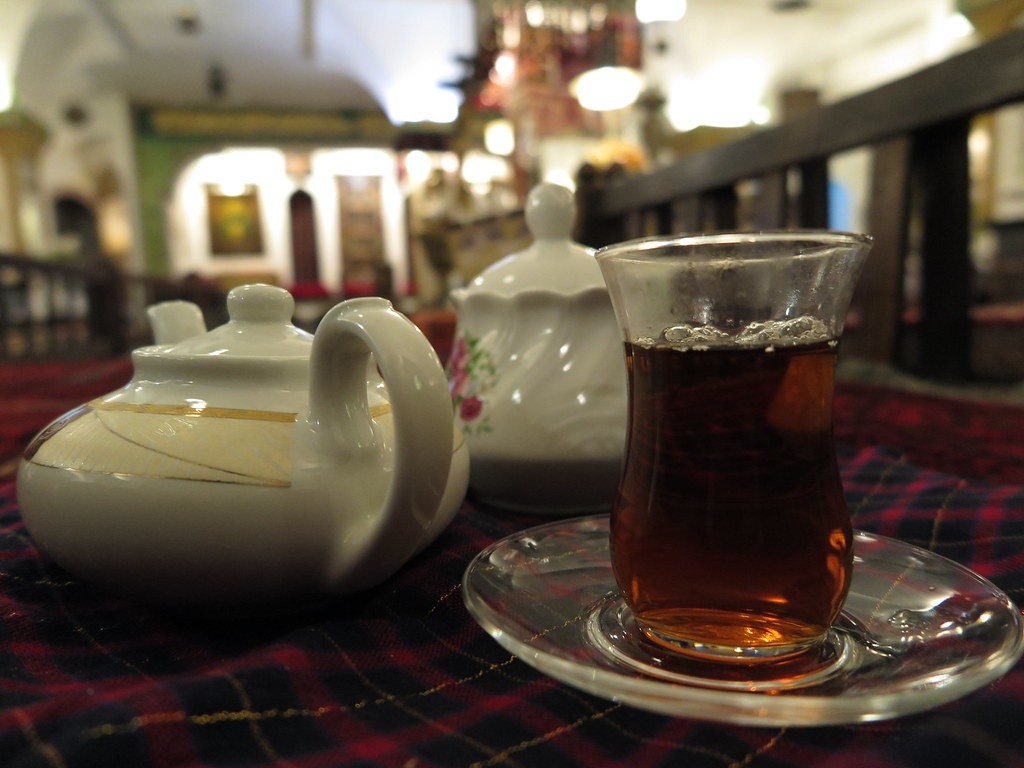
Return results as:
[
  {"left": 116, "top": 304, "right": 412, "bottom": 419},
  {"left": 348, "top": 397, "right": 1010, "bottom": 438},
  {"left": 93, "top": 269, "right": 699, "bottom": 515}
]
[{"left": 592, "top": 228, "right": 877, "bottom": 655}]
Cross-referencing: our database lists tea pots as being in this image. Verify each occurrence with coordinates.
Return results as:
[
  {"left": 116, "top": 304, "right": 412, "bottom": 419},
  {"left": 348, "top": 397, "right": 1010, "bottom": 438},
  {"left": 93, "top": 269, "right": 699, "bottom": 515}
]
[
  {"left": 14, "top": 283, "right": 472, "bottom": 615},
  {"left": 441, "top": 182, "right": 650, "bottom": 516}
]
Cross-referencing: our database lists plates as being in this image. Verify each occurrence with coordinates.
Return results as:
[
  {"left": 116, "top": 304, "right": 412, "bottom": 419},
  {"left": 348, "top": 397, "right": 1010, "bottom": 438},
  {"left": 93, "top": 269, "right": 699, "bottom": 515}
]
[{"left": 462, "top": 511, "right": 1023, "bottom": 727}]
